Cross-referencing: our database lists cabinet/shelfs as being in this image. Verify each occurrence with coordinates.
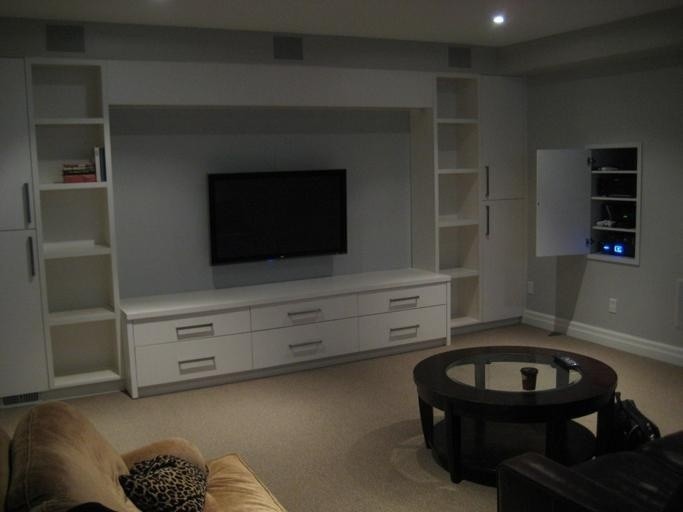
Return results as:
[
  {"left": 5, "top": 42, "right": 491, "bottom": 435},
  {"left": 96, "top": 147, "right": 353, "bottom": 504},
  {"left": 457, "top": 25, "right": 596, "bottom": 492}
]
[
  {"left": 26, "top": 58, "right": 124, "bottom": 401},
  {"left": 477, "top": 76, "right": 528, "bottom": 323},
  {"left": 411, "top": 70, "right": 481, "bottom": 331},
  {"left": 116, "top": 264, "right": 452, "bottom": 401},
  {"left": 534, "top": 144, "right": 641, "bottom": 266},
  {"left": 1, "top": 54, "right": 56, "bottom": 403}
]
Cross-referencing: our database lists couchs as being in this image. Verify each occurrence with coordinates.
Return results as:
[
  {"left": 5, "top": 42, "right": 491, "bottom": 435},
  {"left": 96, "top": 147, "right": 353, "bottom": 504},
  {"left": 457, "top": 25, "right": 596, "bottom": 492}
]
[{"left": 1, "top": 400, "right": 287, "bottom": 512}]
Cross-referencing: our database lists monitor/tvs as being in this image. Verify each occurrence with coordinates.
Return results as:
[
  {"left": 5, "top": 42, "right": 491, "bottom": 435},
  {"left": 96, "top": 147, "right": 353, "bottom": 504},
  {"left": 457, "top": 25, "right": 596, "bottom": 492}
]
[{"left": 207, "top": 169, "right": 348, "bottom": 266}]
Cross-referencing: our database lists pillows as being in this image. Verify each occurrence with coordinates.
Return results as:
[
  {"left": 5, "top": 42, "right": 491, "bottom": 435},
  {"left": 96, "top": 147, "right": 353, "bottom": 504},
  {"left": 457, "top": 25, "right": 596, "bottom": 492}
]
[{"left": 124, "top": 455, "right": 209, "bottom": 508}]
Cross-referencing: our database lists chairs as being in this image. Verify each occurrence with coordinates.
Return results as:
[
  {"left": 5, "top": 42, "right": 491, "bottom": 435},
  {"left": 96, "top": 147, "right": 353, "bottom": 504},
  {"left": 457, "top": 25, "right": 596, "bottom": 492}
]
[{"left": 499, "top": 402, "right": 683, "bottom": 511}]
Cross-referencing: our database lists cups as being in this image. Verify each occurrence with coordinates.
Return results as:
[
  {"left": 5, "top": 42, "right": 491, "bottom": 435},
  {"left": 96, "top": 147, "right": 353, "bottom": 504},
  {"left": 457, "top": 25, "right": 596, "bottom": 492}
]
[{"left": 520, "top": 367, "right": 538, "bottom": 390}]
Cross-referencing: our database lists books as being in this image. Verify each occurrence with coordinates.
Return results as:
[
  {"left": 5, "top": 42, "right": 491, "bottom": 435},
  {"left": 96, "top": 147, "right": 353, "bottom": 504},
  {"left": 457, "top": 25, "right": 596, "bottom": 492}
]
[{"left": 62, "top": 145, "right": 106, "bottom": 182}]
